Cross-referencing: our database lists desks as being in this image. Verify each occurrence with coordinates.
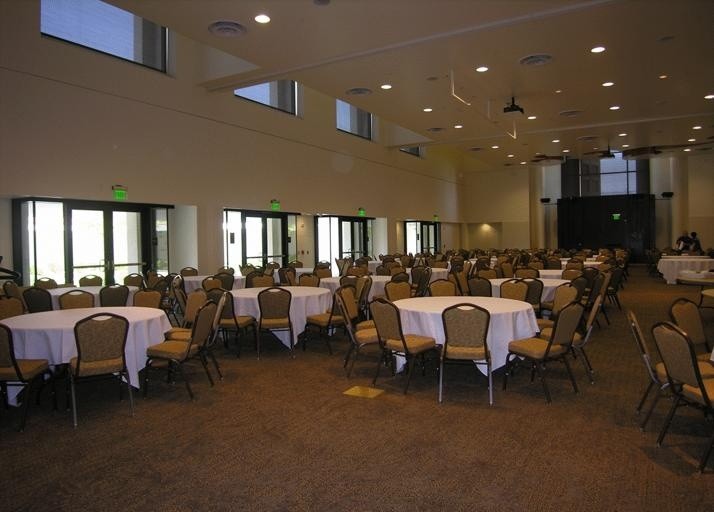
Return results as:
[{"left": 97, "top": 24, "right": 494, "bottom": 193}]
[{"left": 647, "top": 247, "right": 713, "bottom": 308}]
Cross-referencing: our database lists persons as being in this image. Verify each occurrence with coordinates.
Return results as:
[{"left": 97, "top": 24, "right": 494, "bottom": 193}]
[
  {"left": 675, "top": 230, "right": 693, "bottom": 254},
  {"left": 603, "top": 250, "right": 616, "bottom": 266},
  {"left": 688, "top": 231, "right": 704, "bottom": 256},
  {"left": 533, "top": 252, "right": 546, "bottom": 264},
  {"left": 553, "top": 249, "right": 559, "bottom": 256}
]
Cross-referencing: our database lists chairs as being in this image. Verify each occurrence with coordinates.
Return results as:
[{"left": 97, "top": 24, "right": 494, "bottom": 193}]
[
  {"left": 1, "top": 246, "right": 633, "bottom": 360},
  {"left": 67, "top": 312, "right": 136, "bottom": 427},
  {"left": 438, "top": 303, "right": 495, "bottom": 407},
  {"left": 627, "top": 310, "right": 713, "bottom": 431},
  {"left": 369, "top": 296, "right": 440, "bottom": 395},
  {"left": 0, "top": 323, "right": 57, "bottom": 432},
  {"left": 164, "top": 291, "right": 228, "bottom": 381},
  {"left": 669, "top": 296, "right": 713, "bottom": 363},
  {"left": 537, "top": 292, "right": 603, "bottom": 386},
  {"left": 652, "top": 320, "right": 714, "bottom": 475},
  {"left": 334, "top": 293, "right": 384, "bottom": 379},
  {"left": 503, "top": 300, "right": 584, "bottom": 405},
  {"left": 142, "top": 299, "right": 217, "bottom": 401}
]
